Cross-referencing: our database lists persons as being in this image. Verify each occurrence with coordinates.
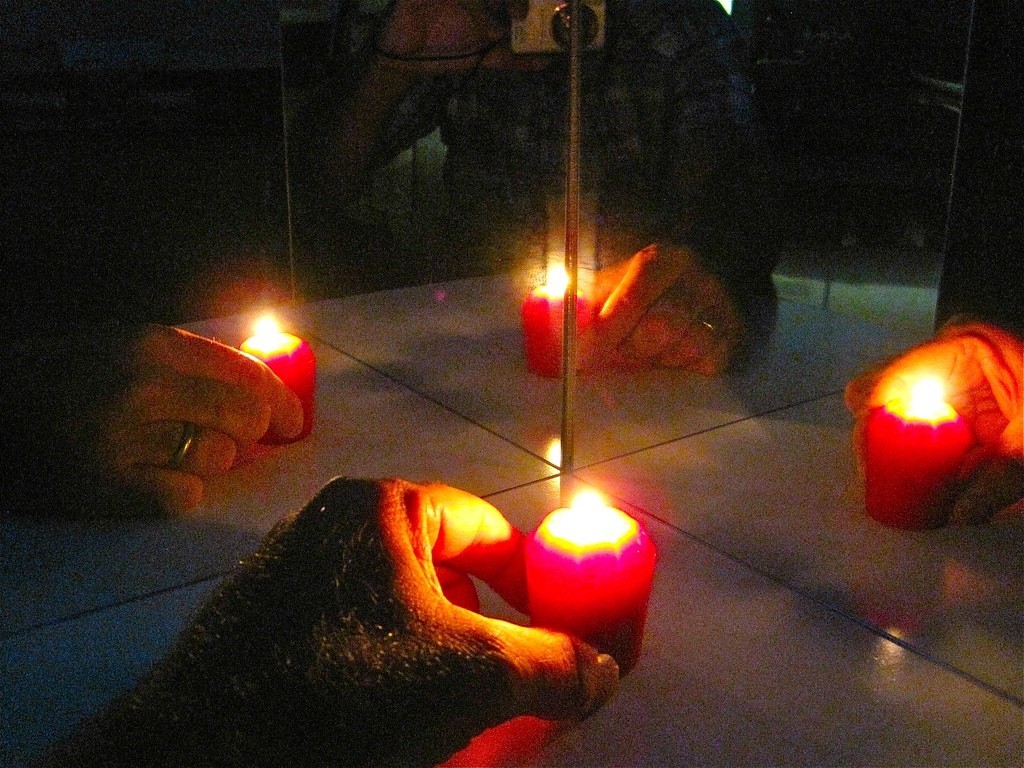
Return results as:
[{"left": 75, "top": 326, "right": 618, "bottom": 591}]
[
  {"left": 317, "top": 0, "right": 777, "bottom": 376},
  {"left": 0, "top": 253, "right": 303, "bottom": 521},
  {"left": 843, "top": 240, "right": 1024, "bottom": 530},
  {"left": 0, "top": 476, "right": 618, "bottom": 768}
]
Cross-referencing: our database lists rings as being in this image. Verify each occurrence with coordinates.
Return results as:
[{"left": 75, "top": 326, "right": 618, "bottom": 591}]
[
  {"left": 166, "top": 422, "right": 195, "bottom": 469},
  {"left": 702, "top": 319, "right": 715, "bottom": 331}
]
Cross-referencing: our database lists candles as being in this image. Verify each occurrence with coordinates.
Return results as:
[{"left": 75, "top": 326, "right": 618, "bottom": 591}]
[
  {"left": 865, "top": 377, "right": 976, "bottom": 534},
  {"left": 521, "top": 264, "right": 592, "bottom": 379},
  {"left": 239, "top": 312, "right": 317, "bottom": 448},
  {"left": 523, "top": 486, "right": 656, "bottom": 680}
]
